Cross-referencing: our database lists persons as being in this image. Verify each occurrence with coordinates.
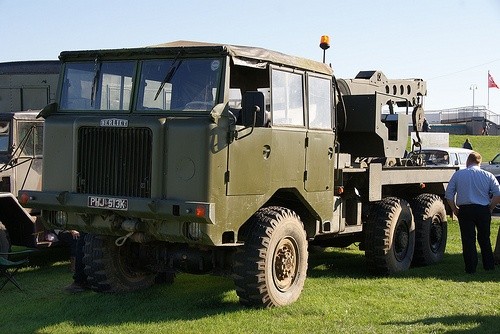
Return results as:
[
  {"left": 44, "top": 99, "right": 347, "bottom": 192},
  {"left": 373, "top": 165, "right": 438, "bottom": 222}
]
[
  {"left": 446, "top": 152, "right": 500, "bottom": 273},
  {"left": 32, "top": 217, "right": 80, "bottom": 272},
  {"left": 66, "top": 232, "right": 88, "bottom": 293},
  {"left": 481, "top": 118, "right": 489, "bottom": 136},
  {"left": 463, "top": 138, "right": 473, "bottom": 149}
]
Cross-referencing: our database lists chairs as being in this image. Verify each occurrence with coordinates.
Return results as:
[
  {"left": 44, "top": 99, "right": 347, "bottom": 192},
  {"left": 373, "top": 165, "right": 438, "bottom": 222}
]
[{"left": 0, "top": 245, "right": 30, "bottom": 293}]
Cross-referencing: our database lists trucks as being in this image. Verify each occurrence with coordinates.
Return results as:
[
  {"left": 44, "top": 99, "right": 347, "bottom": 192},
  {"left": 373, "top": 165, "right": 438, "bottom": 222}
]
[
  {"left": 17, "top": 35, "right": 482, "bottom": 310},
  {"left": 0, "top": 60, "right": 174, "bottom": 270}
]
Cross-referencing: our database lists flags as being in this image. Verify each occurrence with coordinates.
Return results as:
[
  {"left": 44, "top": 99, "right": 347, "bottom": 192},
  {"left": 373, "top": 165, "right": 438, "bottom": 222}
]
[{"left": 487, "top": 72, "right": 500, "bottom": 89}]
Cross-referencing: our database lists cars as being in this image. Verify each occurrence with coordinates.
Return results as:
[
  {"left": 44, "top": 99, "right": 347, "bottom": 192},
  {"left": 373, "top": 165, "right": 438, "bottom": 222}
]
[{"left": 481, "top": 154, "right": 500, "bottom": 185}]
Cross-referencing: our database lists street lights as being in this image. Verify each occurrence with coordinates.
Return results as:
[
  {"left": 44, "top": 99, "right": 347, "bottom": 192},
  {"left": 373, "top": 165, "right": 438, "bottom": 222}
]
[{"left": 470, "top": 84, "right": 478, "bottom": 120}]
